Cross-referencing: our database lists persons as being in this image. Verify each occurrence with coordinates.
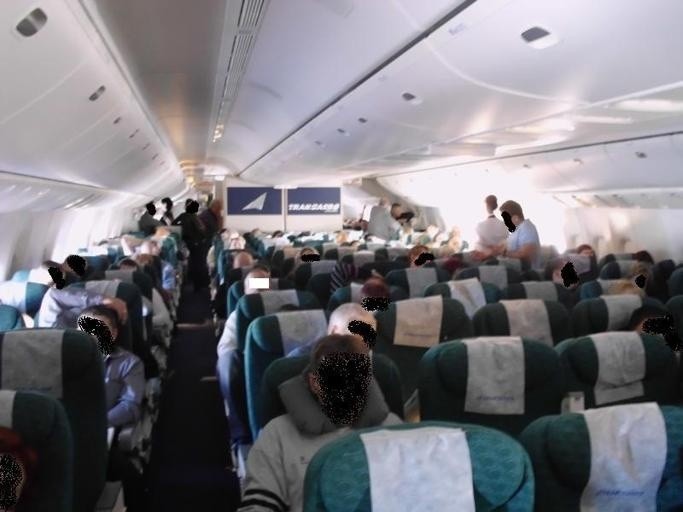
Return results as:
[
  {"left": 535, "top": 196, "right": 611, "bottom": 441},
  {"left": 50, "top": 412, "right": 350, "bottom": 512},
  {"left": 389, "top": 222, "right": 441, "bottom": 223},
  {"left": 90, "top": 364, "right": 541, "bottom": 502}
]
[{"left": 0, "top": 194, "right": 681, "bottom": 512}]
